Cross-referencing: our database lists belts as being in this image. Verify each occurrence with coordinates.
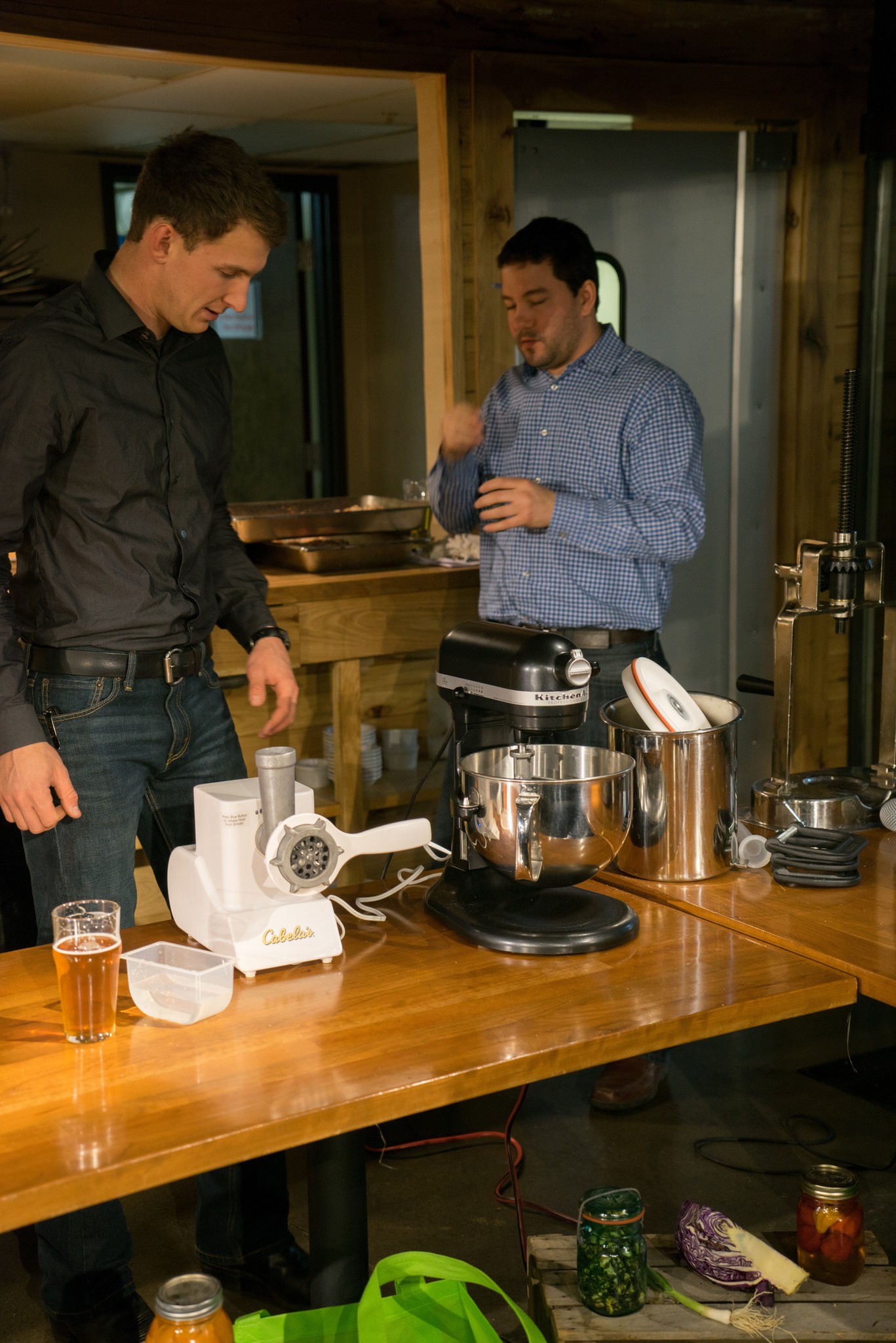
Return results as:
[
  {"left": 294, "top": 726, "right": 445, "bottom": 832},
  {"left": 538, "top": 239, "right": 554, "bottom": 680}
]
[
  {"left": 517, "top": 622, "right": 654, "bottom": 650},
  {"left": 29, "top": 636, "right": 214, "bottom": 685}
]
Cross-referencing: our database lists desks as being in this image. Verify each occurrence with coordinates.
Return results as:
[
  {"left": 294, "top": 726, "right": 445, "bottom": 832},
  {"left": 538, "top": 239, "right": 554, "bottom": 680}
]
[
  {"left": 2, "top": 869, "right": 855, "bottom": 1310},
  {"left": 592, "top": 824, "right": 896, "bottom": 1110}
]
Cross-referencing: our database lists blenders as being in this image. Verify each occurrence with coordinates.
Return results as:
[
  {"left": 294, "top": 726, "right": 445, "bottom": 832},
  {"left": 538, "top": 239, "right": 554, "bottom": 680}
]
[{"left": 424, "top": 619, "right": 641, "bottom": 954}]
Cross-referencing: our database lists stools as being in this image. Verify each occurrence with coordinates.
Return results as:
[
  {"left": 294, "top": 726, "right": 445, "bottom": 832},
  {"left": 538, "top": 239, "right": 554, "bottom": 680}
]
[{"left": 521, "top": 1213, "right": 896, "bottom": 1343}]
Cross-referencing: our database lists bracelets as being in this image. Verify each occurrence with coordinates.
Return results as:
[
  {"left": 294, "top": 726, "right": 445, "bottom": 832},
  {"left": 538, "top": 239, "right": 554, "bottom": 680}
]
[{"left": 247, "top": 626, "right": 292, "bottom": 653}]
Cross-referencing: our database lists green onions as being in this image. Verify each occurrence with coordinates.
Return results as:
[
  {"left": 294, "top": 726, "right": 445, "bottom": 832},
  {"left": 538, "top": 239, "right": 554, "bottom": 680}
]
[{"left": 645, "top": 1264, "right": 799, "bottom": 1343}]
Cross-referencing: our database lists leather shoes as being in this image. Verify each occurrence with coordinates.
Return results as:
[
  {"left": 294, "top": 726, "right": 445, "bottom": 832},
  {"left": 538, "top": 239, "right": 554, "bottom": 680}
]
[
  {"left": 589, "top": 1052, "right": 668, "bottom": 1112},
  {"left": 50, "top": 1243, "right": 314, "bottom": 1343}
]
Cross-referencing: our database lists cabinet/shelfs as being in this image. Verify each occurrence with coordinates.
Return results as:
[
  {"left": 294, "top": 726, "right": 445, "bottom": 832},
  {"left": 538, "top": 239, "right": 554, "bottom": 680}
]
[{"left": 210, "top": 564, "right": 489, "bottom": 849}]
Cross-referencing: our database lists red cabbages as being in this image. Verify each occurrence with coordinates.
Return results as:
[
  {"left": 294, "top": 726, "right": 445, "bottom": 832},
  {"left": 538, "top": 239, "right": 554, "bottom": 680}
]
[{"left": 674, "top": 1199, "right": 811, "bottom": 1307}]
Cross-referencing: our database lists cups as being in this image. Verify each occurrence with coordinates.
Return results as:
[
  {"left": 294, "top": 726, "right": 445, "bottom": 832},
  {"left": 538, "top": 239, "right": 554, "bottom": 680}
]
[
  {"left": 594, "top": 693, "right": 743, "bottom": 884},
  {"left": 49, "top": 899, "right": 123, "bottom": 1045}
]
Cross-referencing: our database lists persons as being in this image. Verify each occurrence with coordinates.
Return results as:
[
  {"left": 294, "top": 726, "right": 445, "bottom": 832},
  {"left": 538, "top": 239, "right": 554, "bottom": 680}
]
[
  {"left": 0, "top": 123, "right": 322, "bottom": 1343},
  {"left": 424, "top": 211, "right": 711, "bottom": 1120}
]
